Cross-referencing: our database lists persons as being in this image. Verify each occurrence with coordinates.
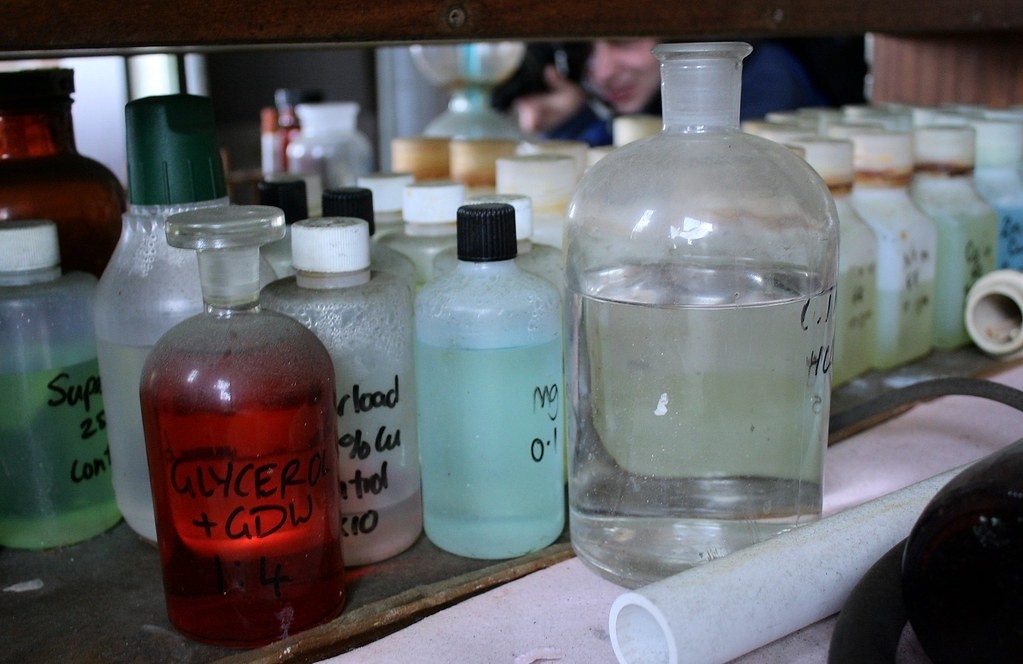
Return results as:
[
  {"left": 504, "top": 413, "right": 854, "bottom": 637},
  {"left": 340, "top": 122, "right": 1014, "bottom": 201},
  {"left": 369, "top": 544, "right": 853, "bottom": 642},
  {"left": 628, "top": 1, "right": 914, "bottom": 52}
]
[{"left": 517, "top": 32, "right": 825, "bottom": 150}]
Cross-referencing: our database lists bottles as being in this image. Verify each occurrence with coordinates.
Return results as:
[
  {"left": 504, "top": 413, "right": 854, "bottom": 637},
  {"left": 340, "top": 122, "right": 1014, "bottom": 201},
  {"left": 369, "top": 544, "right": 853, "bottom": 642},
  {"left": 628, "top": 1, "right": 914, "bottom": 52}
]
[{"left": 1, "top": 40, "right": 1023, "bottom": 653}]
